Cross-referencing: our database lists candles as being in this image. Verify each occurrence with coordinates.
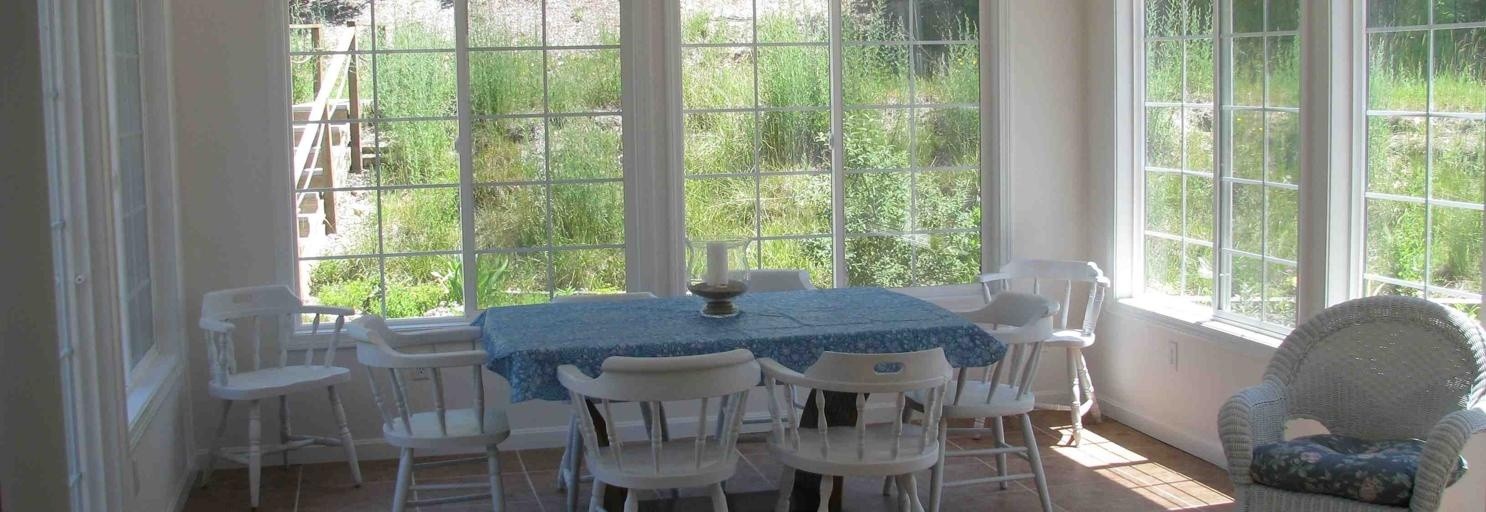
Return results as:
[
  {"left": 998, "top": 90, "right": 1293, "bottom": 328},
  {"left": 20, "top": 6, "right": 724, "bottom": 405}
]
[{"left": 706, "top": 242, "right": 729, "bottom": 285}]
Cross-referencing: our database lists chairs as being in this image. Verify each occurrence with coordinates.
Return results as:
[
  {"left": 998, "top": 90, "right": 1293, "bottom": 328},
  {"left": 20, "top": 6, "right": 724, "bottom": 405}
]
[
  {"left": 971, "top": 258, "right": 1109, "bottom": 448},
  {"left": 555, "top": 347, "right": 762, "bottom": 512},
  {"left": 349, "top": 309, "right": 512, "bottom": 512},
  {"left": 1214, "top": 294, "right": 1486, "bottom": 512},
  {"left": 191, "top": 283, "right": 371, "bottom": 505},
  {"left": 754, "top": 347, "right": 955, "bottom": 512},
  {"left": 882, "top": 288, "right": 1062, "bottom": 511},
  {"left": 716, "top": 267, "right": 822, "bottom": 463}
]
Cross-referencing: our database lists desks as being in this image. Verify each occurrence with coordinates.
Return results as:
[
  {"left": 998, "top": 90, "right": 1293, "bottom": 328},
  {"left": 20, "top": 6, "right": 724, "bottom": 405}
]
[{"left": 474, "top": 283, "right": 1008, "bottom": 511}]
